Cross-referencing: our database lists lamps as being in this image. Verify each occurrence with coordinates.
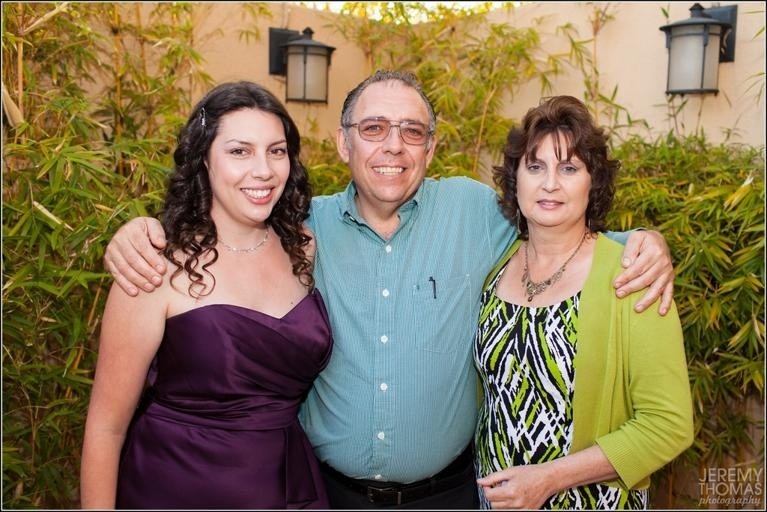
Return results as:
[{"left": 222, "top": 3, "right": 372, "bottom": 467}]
[
  {"left": 270, "top": 25, "right": 336, "bottom": 106},
  {"left": 659, "top": 3, "right": 737, "bottom": 95}
]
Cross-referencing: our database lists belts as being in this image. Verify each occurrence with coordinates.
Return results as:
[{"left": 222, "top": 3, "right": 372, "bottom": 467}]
[{"left": 321, "top": 460, "right": 474, "bottom": 507}]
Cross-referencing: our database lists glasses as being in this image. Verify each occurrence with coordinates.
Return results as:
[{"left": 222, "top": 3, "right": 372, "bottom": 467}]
[{"left": 346, "top": 117, "right": 435, "bottom": 145}]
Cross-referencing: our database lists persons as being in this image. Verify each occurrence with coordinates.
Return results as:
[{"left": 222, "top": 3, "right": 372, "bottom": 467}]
[
  {"left": 79, "top": 82, "right": 333, "bottom": 510},
  {"left": 104, "top": 69, "right": 676, "bottom": 508},
  {"left": 472, "top": 94, "right": 694, "bottom": 509}
]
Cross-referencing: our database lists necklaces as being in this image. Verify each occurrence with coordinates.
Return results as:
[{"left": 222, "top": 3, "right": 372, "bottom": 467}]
[
  {"left": 217, "top": 225, "right": 268, "bottom": 253},
  {"left": 521, "top": 234, "right": 587, "bottom": 302}
]
